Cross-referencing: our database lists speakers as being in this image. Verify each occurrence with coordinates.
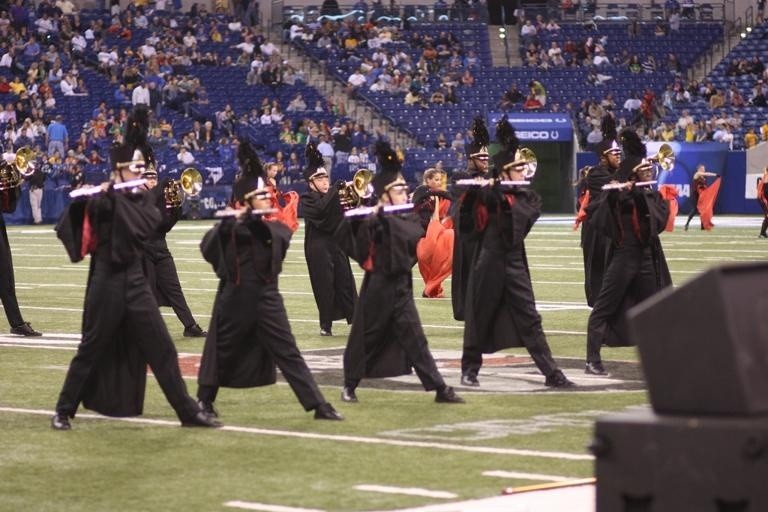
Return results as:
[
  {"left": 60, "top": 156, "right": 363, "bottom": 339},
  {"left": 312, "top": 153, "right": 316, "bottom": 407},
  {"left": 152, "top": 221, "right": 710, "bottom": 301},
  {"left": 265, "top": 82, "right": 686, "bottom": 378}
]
[
  {"left": 624, "top": 260, "right": 768, "bottom": 415},
  {"left": 587, "top": 413, "right": 767, "bottom": 512}
]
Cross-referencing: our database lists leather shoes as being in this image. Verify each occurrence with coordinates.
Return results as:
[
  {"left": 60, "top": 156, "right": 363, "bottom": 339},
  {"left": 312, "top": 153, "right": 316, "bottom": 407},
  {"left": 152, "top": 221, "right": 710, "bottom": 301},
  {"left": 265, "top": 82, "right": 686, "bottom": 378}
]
[
  {"left": 180, "top": 399, "right": 225, "bottom": 429},
  {"left": 584, "top": 360, "right": 611, "bottom": 377},
  {"left": 321, "top": 328, "right": 333, "bottom": 337},
  {"left": 434, "top": 388, "right": 465, "bottom": 403},
  {"left": 544, "top": 370, "right": 576, "bottom": 388},
  {"left": 51, "top": 413, "right": 70, "bottom": 429},
  {"left": 182, "top": 324, "right": 208, "bottom": 338},
  {"left": 340, "top": 386, "right": 358, "bottom": 403},
  {"left": 460, "top": 371, "right": 480, "bottom": 386},
  {"left": 11, "top": 323, "right": 42, "bottom": 338},
  {"left": 313, "top": 402, "right": 342, "bottom": 421}
]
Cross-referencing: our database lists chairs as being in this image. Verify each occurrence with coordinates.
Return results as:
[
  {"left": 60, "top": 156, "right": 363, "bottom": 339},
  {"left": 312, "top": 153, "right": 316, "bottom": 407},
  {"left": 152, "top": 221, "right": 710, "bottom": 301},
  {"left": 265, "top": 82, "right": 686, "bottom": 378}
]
[{"left": 1, "top": 1, "right": 768, "bottom": 187}]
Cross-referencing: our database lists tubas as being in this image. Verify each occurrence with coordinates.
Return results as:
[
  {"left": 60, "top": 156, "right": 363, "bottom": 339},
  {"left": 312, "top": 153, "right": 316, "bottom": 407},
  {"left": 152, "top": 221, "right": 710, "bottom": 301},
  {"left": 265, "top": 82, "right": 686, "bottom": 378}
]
[
  {"left": 163, "top": 167, "right": 205, "bottom": 209},
  {"left": 338, "top": 168, "right": 374, "bottom": 212},
  {"left": 644, "top": 143, "right": 676, "bottom": 180},
  {"left": 0, "top": 148, "right": 37, "bottom": 190}
]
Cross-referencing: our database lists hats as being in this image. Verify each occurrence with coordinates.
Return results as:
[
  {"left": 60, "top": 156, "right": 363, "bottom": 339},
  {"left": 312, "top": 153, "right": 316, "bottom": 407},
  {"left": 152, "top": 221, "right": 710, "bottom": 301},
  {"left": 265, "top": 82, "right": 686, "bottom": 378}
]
[
  {"left": 304, "top": 162, "right": 329, "bottom": 179},
  {"left": 371, "top": 170, "right": 409, "bottom": 192},
  {"left": 140, "top": 145, "right": 157, "bottom": 178},
  {"left": 624, "top": 155, "right": 655, "bottom": 172},
  {"left": 494, "top": 147, "right": 527, "bottom": 171},
  {"left": 467, "top": 140, "right": 491, "bottom": 160},
  {"left": 234, "top": 168, "right": 271, "bottom": 201},
  {"left": 597, "top": 138, "right": 622, "bottom": 155},
  {"left": 111, "top": 144, "right": 144, "bottom": 173}
]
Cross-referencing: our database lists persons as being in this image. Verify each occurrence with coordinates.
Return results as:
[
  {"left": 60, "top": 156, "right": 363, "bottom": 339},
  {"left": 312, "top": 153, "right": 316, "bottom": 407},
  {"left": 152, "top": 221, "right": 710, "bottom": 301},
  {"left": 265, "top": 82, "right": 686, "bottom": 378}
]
[
  {"left": 1, "top": 1, "right": 768, "bottom": 236},
  {"left": 574, "top": 113, "right": 678, "bottom": 377},
  {"left": 51, "top": 105, "right": 579, "bottom": 429},
  {"left": 1, "top": 147, "right": 43, "bottom": 337}
]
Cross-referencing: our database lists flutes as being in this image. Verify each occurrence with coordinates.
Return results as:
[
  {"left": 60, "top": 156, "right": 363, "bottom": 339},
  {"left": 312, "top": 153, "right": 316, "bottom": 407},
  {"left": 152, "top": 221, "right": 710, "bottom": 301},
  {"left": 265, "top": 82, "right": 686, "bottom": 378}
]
[
  {"left": 214, "top": 207, "right": 279, "bottom": 217},
  {"left": 601, "top": 181, "right": 658, "bottom": 191},
  {"left": 69, "top": 179, "right": 146, "bottom": 199},
  {"left": 344, "top": 203, "right": 414, "bottom": 218},
  {"left": 455, "top": 179, "right": 532, "bottom": 187}
]
